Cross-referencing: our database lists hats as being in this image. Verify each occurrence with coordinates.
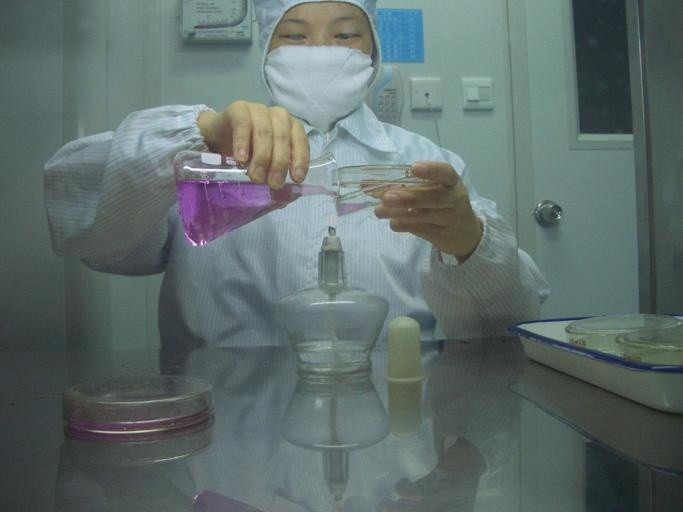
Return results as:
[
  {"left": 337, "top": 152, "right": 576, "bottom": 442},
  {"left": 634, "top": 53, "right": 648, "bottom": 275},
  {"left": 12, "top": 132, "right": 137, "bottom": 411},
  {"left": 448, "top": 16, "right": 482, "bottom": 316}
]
[{"left": 252, "top": 1, "right": 381, "bottom": 83}]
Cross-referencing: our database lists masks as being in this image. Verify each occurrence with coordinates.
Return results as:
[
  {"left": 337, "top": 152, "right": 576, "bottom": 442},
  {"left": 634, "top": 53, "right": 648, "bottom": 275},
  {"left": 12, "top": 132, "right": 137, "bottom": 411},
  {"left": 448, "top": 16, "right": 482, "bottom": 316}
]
[{"left": 264, "top": 45, "right": 374, "bottom": 131}]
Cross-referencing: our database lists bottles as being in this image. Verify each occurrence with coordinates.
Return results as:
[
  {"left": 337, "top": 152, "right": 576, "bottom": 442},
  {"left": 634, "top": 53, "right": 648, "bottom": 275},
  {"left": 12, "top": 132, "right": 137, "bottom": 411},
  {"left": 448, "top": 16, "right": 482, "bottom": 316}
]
[
  {"left": 276, "top": 250, "right": 390, "bottom": 387},
  {"left": 280, "top": 372, "right": 395, "bottom": 486},
  {"left": 171, "top": 149, "right": 340, "bottom": 249}
]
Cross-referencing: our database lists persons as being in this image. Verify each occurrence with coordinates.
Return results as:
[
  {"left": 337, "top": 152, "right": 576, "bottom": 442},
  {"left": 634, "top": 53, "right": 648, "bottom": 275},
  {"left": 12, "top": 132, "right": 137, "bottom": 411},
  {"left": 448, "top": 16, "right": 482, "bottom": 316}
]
[
  {"left": 136, "top": 353, "right": 524, "bottom": 510},
  {"left": 43, "top": 1, "right": 552, "bottom": 352}
]
